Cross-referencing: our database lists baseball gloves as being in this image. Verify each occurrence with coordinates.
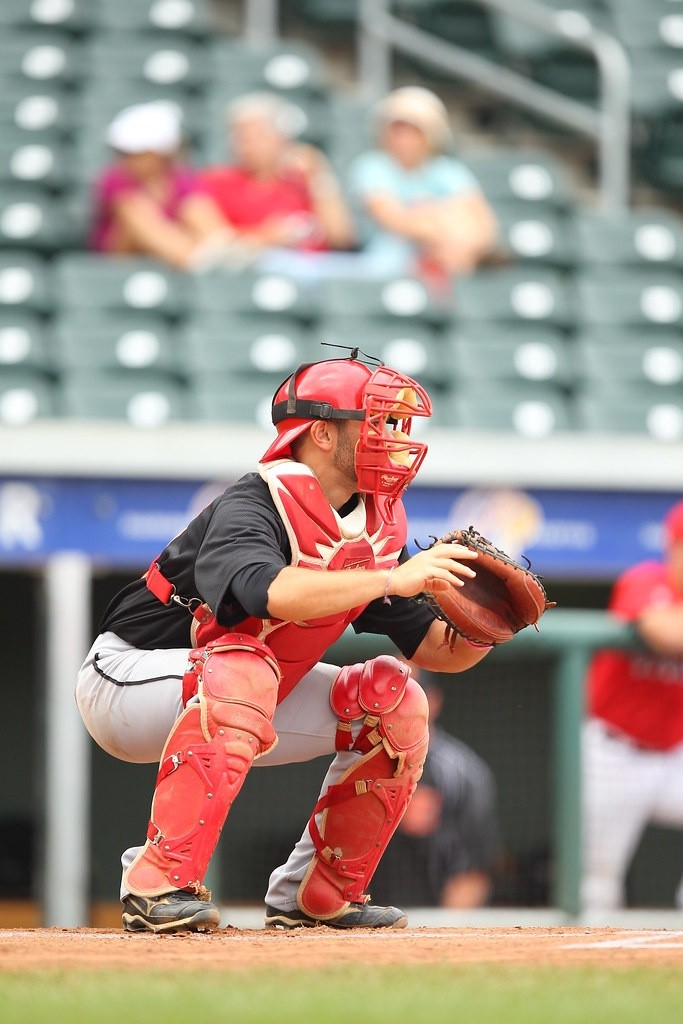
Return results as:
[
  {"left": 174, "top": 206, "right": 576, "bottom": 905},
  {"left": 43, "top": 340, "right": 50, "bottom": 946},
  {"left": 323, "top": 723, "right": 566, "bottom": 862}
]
[{"left": 413, "top": 525, "right": 556, "bottom": 654}]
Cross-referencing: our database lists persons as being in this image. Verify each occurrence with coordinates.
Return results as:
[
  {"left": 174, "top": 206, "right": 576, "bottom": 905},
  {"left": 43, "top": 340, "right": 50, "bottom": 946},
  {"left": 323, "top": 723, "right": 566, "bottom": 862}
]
[
  {"left": 92, "top": 96, "right": 268, "bottom": 275},
  {"left": 74, "top": 343, "right": 496, "bottom": 937},
  {"left": 574, "top": 496, "right": 683, "bottom": 925},
  {"left": 343, "top": 86, "right": 499, "bottom": 275},
  {"left": 199, "top": 89, "right": 355, "bottom": 256},
  {"left": 364, "top": 656, "right": 500, "bottom": 909}
]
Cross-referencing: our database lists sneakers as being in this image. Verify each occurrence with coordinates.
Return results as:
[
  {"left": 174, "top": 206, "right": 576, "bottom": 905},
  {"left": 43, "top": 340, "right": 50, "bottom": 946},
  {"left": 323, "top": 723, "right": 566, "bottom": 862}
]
[
  {"left": 125, "top": 887, "right": 219, "bottom": 934},
  {"left": 264, "top": 901, "right": 408, "bottom": 930}
]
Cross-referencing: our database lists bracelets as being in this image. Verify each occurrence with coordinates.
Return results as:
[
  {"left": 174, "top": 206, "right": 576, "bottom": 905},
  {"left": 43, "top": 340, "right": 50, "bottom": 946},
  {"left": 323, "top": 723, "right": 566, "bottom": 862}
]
[{"left": 383, "top": 563, "right": 397, "bottom": 604}]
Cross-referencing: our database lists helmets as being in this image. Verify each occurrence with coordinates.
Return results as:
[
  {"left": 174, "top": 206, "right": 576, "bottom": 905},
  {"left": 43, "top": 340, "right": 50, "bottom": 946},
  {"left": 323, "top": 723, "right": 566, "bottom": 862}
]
[{"left": 258, "top": 358, "right": 433, "bottom": 524}]
